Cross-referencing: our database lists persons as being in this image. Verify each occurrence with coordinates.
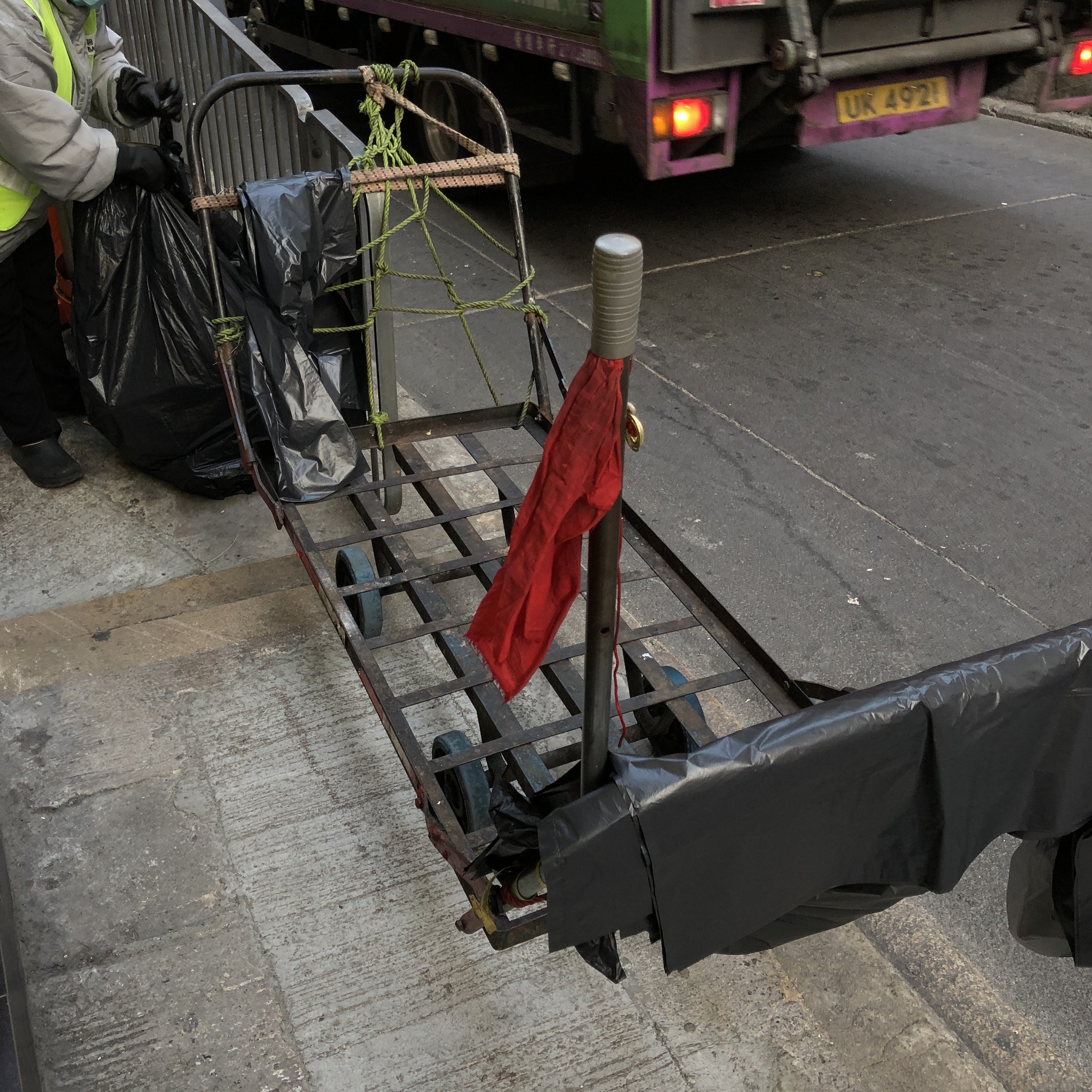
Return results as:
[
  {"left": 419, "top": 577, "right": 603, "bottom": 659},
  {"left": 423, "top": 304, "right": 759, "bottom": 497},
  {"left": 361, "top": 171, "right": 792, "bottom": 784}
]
[{"left": 0, "top": 0, "right": 182, "bottom": 489}]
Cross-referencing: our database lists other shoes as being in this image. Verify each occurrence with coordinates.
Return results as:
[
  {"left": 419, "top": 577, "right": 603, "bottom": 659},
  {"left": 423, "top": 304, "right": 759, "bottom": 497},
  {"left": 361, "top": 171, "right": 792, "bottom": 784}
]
[
  {"left": 10, "top": 439, "right": 84, "bottom": 489},
  {"left": 55, "top": 370, "right": 86, "bottom": 418}
]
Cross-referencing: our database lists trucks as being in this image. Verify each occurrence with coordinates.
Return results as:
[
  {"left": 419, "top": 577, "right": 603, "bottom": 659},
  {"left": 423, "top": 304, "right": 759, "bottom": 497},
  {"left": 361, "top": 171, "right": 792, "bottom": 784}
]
[{"left": 289, "top": 0, "right": 1070, "bottom": 215}]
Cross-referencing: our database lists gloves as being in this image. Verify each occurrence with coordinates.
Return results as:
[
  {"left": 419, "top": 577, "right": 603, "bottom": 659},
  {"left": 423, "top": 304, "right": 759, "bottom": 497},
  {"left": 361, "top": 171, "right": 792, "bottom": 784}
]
[
  {"left": 114, "top": 142, "right": 175, "bottom": 195},
  {"left": 117, "top": 67, "right": 183, "bottom": 123}
]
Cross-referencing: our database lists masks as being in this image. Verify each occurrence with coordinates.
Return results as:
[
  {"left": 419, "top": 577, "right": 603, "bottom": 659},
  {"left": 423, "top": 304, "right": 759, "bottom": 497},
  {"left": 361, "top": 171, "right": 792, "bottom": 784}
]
[{"left": 72, "top": 0, "right": 111, "bottom": 9}]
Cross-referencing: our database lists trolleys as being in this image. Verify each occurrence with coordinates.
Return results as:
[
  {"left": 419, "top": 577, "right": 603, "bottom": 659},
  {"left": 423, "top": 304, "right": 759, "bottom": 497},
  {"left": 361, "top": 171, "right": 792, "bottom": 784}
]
[{"left": 186, "top": 67, "right": 868, "bottom": 984}]
